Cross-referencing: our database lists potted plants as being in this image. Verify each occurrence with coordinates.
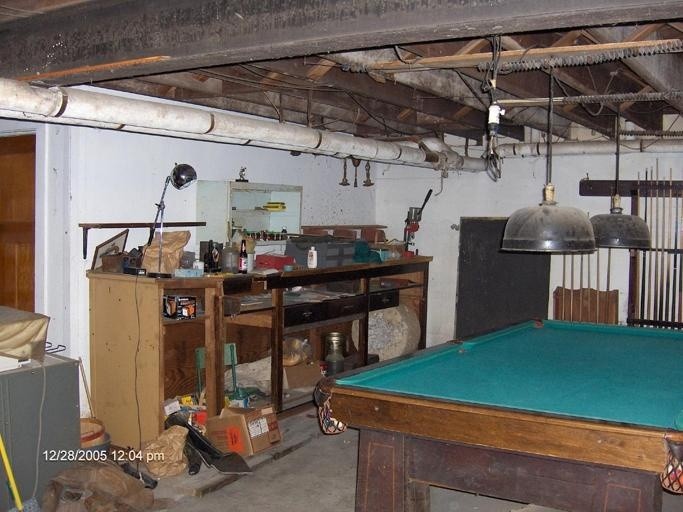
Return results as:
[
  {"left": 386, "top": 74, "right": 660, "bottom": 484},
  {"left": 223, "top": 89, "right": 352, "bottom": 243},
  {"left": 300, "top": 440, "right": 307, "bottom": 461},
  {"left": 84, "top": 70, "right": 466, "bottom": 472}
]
[{"left": 91, "top": 229, "right": 129, "bottom": 270}]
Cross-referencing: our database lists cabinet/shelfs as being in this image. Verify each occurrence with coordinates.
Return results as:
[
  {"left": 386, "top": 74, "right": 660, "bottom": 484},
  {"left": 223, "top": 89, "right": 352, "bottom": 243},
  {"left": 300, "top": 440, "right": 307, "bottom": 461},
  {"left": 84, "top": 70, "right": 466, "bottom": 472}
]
[
  {"left": 220, "top": 226, "right": 287, "bottom": 274},
  {"left": 307, "top": 246, "right": 317, "bottom": 268}
]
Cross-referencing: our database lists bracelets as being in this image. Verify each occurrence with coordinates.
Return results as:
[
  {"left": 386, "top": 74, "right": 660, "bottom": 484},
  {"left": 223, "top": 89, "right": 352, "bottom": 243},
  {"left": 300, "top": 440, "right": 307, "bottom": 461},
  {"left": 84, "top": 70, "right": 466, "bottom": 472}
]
[{"left": 319, "top": 362, "right": 327, "bottom": 377}]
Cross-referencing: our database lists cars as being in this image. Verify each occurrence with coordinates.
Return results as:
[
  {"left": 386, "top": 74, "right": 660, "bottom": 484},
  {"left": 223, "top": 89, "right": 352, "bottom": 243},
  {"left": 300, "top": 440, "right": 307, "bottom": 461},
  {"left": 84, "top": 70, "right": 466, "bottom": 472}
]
[{"left": 193, "top": 260, "right": 204, "bottom": 276}]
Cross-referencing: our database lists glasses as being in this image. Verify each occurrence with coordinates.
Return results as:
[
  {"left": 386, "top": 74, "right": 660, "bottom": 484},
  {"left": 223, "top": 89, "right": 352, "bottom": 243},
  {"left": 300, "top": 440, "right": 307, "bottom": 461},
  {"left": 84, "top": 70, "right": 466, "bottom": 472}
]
[
  {"left": 590, "top": 101, "right": 652, "bottom": 251},
  {"left": 147, "top": 163, "right": 197, "bottom": 278},
  {"left": 501, "top": 67, "right": 596, "bottom": 255}
]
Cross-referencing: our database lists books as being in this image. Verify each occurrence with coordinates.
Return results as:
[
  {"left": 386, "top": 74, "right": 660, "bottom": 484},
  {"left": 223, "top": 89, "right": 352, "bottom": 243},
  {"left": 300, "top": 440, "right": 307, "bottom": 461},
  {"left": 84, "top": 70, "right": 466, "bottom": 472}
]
[
  {"left": 206, "top": 405, "right": 281, "bottom": 459},
  {"left": 163, "top": 294, "right": 198, "bottom": 321}
]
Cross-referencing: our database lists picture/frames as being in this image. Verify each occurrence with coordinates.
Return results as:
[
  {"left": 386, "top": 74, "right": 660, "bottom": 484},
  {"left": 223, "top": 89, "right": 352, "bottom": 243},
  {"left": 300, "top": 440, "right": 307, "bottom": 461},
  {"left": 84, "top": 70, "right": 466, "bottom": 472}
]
[{"left": 315, "top": 318, "right": 683, "bottom": 512}]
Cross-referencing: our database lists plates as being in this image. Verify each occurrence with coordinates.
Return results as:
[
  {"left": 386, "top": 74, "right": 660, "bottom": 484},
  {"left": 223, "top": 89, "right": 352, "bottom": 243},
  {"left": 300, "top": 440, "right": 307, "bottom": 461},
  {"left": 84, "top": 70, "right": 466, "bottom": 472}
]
[
  {"left": 366, "top": 255, "right": 433, "bottom": 365},
  {"left": 87, "top": 265, "right": 216, "bottom": 454},
  {"left": 0, "top": 348, "right": 80, "bottom": 512},
  {"left": 197, "top": 179, "right": 303, "bottom": 261},
  {"left": 216, "top": 273, "right": 278, "bottom": 420},
  {"left": 278, "top": 263, "right": 366, "bottom": 420}
]
